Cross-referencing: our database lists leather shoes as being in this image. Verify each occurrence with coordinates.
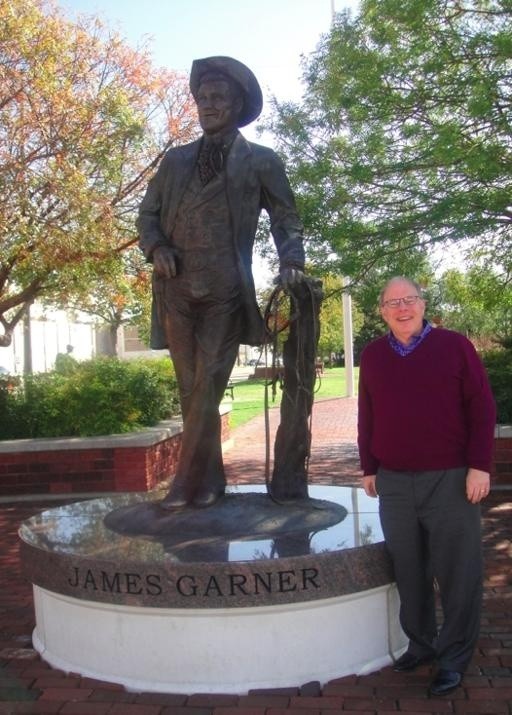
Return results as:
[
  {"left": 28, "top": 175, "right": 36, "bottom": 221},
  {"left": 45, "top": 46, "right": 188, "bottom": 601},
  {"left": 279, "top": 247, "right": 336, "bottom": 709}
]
[
  {"left": 428, "top": 669, "right": 465, "bottom": 698},
  {"left": 391, "top": 650, "right": 438, "bottom": 674}
]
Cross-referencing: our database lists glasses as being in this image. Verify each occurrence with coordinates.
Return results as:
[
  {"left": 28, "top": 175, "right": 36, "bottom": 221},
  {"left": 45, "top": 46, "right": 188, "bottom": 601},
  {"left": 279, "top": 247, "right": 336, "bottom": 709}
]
[{"left": 380, "top": 295, "right": 421, "bottom": 309}]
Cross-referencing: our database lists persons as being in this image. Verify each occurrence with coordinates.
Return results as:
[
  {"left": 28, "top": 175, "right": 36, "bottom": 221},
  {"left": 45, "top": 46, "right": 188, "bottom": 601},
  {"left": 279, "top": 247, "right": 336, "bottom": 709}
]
[
  {"left": 133, "top": 55, "right": 305, "bottom": 511},
  {"left": 356, "top": 275, "right": 498, "bottom": 697}
]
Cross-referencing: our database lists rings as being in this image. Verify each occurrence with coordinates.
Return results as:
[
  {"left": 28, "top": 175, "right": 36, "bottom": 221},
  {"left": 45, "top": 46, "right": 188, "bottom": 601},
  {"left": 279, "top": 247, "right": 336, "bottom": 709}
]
[{"left": 479, "top": 489, "right": 486, "bottom": 494}]
